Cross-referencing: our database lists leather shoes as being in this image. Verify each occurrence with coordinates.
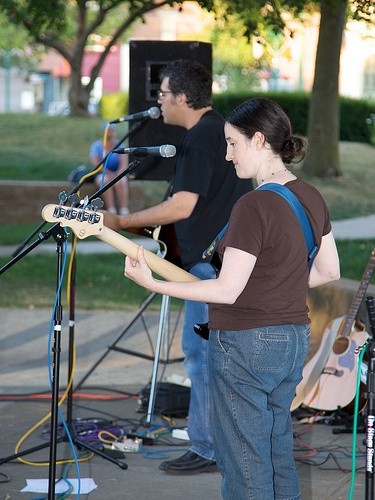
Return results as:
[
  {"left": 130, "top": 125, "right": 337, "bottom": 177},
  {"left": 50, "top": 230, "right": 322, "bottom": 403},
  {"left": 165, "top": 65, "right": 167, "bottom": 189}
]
[{"left": 159, "top": 450, "right": 218, "bottom": 475}]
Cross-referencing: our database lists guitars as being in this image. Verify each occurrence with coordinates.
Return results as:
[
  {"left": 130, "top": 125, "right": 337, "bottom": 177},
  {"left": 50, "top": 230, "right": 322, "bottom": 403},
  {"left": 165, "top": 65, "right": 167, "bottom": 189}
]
[
  {"left": 41, "top": 190, "right": 201, "bottom": 282},
  {"left": 114, "top": 224, "right": 188, "bottom": 272},
  {"left": 292, "top": 244, "right": 375, "bottom": 417}
]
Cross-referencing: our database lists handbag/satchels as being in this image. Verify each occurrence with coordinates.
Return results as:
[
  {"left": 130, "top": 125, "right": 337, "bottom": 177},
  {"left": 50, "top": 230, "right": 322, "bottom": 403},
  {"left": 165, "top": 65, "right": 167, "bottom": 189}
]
[{"left": 67, "top": 165, "right": 95, "bottom": 184}]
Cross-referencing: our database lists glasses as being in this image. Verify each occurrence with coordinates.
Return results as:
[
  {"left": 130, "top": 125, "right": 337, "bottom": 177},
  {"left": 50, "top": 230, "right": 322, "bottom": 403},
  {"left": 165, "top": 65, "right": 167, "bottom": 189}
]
[{"left": 156, "top": 88, "right": 172, "bottom": 97}]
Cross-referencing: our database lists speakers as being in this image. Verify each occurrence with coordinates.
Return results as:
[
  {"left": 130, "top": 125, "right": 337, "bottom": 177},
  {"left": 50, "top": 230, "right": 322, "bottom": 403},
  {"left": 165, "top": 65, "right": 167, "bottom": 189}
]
[{"left": 128, "top": 39, "right": 214, "bottom": 180}]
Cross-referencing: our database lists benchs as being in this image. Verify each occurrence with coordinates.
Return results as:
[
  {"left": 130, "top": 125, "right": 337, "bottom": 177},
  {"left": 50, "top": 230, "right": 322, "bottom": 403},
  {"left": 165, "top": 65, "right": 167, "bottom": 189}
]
[{"left": 0, "top": 180, "right": 145, "bottom": 223}]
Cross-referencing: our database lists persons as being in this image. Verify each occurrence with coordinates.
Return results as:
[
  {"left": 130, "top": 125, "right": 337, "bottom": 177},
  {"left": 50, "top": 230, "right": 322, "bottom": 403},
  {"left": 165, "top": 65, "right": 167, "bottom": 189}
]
[
  {"left": 125, "top": 99, "right": 341, "bottom": 500},
  {"left": 97, "top": 60, "right": 255, "bottom": 475},
  {"left": 90, "top": 122, "right": 129, "bottom": 215}
]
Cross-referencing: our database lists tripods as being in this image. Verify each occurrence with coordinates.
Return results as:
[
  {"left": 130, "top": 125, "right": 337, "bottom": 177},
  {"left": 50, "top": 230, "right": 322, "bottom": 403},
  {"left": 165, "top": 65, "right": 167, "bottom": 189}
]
[{"left": 0, "top": 118, "right": 150, "bottom": 471}]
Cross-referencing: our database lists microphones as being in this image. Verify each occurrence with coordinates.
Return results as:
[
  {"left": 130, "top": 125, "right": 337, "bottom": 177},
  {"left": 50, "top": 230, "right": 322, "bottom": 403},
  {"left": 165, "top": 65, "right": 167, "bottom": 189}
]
[
  {"left": 110, "top": 145, "right": 177, "bottom": 157},
  {"left": 110, "top": 107, "right": 161, "bottom": 125}
]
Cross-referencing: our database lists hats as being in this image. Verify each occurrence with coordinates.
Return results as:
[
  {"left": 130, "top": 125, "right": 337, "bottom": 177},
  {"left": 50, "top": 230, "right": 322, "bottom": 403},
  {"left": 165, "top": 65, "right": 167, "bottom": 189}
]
[{"left": 102, "top": 121, "right": 115, "bottom": 128}]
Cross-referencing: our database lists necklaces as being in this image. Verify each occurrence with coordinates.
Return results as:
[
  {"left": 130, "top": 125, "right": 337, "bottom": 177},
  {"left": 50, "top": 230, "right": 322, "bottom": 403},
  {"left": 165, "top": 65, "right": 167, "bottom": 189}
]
[{"left": 258, "top": 168, "right": 289, "bottom": 186}]
